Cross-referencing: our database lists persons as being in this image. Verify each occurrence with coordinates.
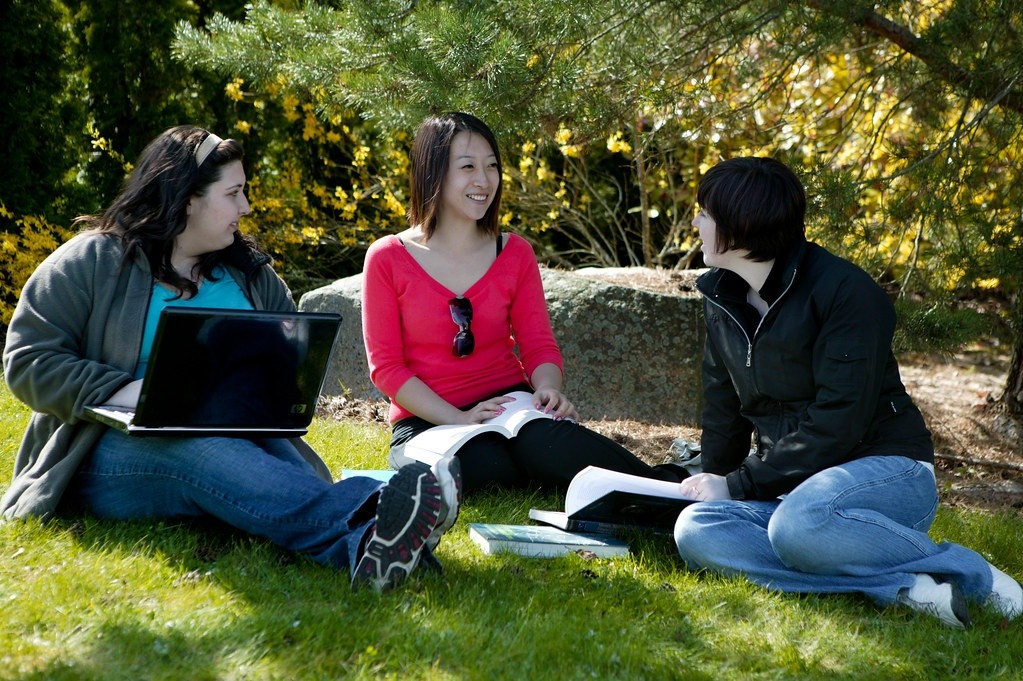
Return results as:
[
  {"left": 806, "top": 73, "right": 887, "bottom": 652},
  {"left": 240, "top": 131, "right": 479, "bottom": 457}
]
[
  {"left": 361, "top": 112, "right": 691, "bottom": 530},
  {"left": 1, "top": 125, "right": 462, "bottom": 597},
  {"left": 672, "top": 156, "right": 1023, "bottom": 633}
]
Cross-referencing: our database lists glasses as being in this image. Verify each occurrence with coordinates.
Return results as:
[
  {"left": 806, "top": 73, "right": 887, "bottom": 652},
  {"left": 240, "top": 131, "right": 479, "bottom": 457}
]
[{"left": 449, "top": 295, "right": 475, "bottom": 357}]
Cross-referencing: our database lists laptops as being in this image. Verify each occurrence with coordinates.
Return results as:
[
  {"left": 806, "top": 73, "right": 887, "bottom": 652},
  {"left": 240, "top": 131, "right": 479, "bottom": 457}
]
[{"left": 81, "top": 305, "right": 343, "bottom": 436}]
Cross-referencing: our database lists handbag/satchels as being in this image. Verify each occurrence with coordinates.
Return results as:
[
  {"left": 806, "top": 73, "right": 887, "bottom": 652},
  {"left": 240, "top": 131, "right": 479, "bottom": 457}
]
[{"left": 663, "top": 438, "right": 703, "bottom": 475}]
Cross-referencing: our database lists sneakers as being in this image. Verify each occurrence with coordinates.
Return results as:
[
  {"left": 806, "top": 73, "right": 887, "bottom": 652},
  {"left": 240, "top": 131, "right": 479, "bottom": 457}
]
[
  {"left": 973, "top": 560, "right": 1023, "bottom": 622},
  {"left": 896, "top": 573, "right": 969, "bottom": 629},
  {"left": 426, "top": 454, "right": 462, "bottom": 553},
  {"left": 349, "top": 461, "right": 443, "bottom": 593}
]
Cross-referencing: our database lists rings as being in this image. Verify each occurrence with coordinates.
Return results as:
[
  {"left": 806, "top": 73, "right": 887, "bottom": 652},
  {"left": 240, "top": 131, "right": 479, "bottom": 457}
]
[{"left": 692, "top": 487, "right": 700, "bottom": 494}]
